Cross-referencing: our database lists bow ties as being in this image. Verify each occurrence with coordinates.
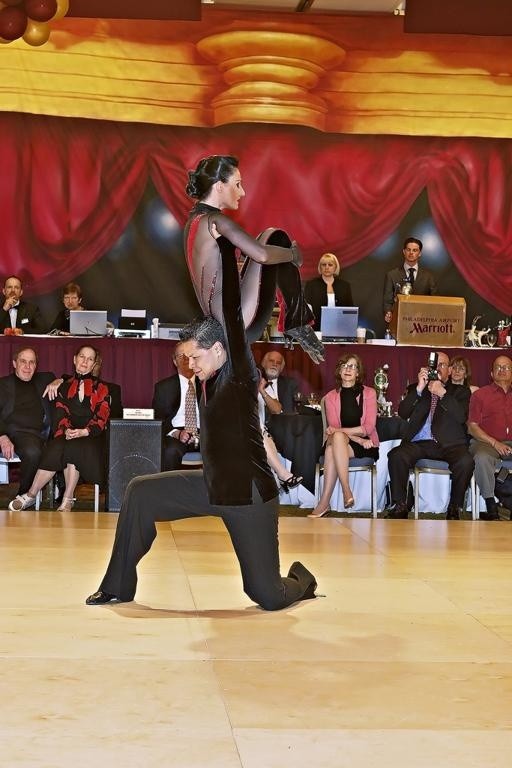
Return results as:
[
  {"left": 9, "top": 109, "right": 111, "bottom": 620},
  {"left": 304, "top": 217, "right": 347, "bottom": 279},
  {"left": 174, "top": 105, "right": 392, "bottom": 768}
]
[{"left": 9, "top": 305, "right": 18, "bottom": 309}]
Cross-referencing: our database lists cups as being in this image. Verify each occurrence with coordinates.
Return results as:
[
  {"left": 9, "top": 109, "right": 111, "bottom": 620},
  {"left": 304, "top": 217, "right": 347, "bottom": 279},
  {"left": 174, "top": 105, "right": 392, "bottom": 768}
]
[
  {"left": 356, "top": 328, "right": 366, "bottom": 344},
  {"left": 308, "top": 393, "right": 319, "bottom": 406},
  {"left": 151, "top": 324, "right": 159, "bottom": 338},
  {"left": 487, "top": 333, "right": 497, "bottom": 347},
  {"left": 386, "top": 406, "right": 391, "bottom": 417},
  {"left": 498, "top": 329, "right": 508, "bottom": 345},
  {"left": 314, "top": 331, "right": 323, "bottom": 342}
]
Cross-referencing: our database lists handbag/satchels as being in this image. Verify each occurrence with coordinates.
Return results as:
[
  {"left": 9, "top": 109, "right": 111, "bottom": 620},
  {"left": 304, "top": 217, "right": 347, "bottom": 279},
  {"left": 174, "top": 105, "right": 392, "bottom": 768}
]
[{"left": 385, "top": 479, "right": 415, "bottom": 512}]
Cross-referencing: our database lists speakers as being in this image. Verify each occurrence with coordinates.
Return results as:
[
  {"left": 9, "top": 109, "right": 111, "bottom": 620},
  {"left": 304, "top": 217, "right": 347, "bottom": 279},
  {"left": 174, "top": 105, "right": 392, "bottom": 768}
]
[{"left": 109, "top": 417, "right": 162, "bottom": 513}]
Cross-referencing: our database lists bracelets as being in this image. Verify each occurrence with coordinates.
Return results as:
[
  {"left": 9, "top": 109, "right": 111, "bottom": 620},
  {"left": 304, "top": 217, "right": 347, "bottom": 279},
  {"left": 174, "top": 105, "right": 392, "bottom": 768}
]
[
  {"left": 263, "top": 392, "right": 269, "bottom": 398},
  {"left": 492, "top": 438, "right": 497, "bottom": 447}
]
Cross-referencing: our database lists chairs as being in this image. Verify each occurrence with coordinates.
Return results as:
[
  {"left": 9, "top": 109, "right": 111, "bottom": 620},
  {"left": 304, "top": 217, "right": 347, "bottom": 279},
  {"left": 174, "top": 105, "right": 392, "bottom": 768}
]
[
  {"left": 182, "top": 451, "right": 206, "bottom": 468},
  {"left": 409, "top": 459, "right": 477, "bottom": 520},
  {"left": 315, "top": 456, "right": 378, "bottom": 518}
]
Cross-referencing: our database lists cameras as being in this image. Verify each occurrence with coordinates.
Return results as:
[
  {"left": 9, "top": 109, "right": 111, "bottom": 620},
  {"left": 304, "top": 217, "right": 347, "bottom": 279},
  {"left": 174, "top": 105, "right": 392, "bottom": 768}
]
[
  {"left": 427, "top": 352, "right": 438, "bottom": 381},
  {"left": 193, "top": 434, "right": 200, "bottom": 449}
]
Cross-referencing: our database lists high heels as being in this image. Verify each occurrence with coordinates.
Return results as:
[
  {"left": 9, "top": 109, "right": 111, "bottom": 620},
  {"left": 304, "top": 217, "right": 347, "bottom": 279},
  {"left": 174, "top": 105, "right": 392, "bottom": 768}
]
[
  {"left": 307, "top": 505, "right": 331, "bottom": 518},
  {"left": 278, "top": 474, "right": 304, "bottom": 493},
  {"left": 344, "top": 498, "right": 355, "bottom": 509}
]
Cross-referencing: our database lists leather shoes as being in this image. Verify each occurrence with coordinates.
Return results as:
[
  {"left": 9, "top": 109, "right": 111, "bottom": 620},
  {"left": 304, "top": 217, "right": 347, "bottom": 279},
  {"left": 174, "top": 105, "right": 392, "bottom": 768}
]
[
  {"left": 447, "top": 509, "right": 460, "bottom": 520},
  {"left": 488, "top": 510, "right": 500, "bottom": 520},
  {"left": 384, "top": 506, "right": 409, "bottom": 517},
  {"left": 86, "top": 591, "right": 116, "bottom": 606},
  {"left": 286, "top": 324, "right": 326, "bottom": 364}
]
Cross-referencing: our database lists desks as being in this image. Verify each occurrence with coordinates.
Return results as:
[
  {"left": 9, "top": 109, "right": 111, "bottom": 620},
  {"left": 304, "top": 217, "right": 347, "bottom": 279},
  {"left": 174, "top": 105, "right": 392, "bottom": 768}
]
[
  {"left": 270, "top": 407, "right": 321, "bottom": 476},
  {"left": 376, "top": 410, "right": 406, "bottom": 441},
  {"left": 1, "top": 332, "right": 512, "bottom": 449}
]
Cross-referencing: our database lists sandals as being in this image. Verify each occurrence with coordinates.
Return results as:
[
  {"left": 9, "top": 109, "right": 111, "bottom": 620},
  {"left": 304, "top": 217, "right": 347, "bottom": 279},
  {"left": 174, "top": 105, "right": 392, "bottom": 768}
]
[
  {"left": 56, "top": 496, "right": 76, "bottom": 512},
  {"left": 7, "top": 492, "right": 36, "bottom": 511}
]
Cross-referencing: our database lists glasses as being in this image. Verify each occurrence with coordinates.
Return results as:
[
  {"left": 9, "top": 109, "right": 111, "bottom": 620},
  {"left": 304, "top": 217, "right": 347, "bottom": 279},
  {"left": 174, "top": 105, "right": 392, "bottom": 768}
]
[
  {"left": 436, "top": 363, "right": 449, "bottom": 369},
  {"left": 340, "top": 364, "right": 359, "bottom": 370},
  {"left": 492, "top": 365, "right": 512, "bottom": 372}
]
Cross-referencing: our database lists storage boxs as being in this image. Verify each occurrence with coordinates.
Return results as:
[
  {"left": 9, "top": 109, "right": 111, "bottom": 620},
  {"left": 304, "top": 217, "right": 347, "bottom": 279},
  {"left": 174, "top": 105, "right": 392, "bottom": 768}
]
[{"left": 388, "top": 289, "right": 467, "bottom": 349}]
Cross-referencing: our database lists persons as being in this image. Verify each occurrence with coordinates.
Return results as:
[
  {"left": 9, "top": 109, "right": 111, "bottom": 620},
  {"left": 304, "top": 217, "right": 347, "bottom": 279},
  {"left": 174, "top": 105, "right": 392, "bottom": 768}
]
[
  {"left": 383, "top": 237, "right": 438, "bottom": 340},
  {"left": 182, "top": 154, "right": 326, "bottom": 365},
  {"left": 304, "top": 253, "right": 366, "bottom": 342},
  {"left": 151, "top": 342, "right": 201, "bottom": 471},
  {"left": 86, "top": 221, "right": 327, "bottom": 611},
  {"left": 1, "top": 347, "right": 73, "bottom": 510},
  {"left": 255, "top": 351, "right": 300, "bottom": 413},
  {"left": 8, "top": 344, "right": 110, "bottom": 511},
  {"left": 466, "top": 356, "right": 512, "bottom": 520},
  {"left": 447, "top": 357, "right": 480, "bottom": 394},
  {"left": 254, "top": 368, "right": 303, "bottom": 493},
  {"left": 49, "top": 284, "right": 89, "bottom": 335},
  {"left": 387, "top": 352, "right": 474, "bottom": 519},
  {"left": 0, "top": 277, "right": 47, "bottom": 334},
  {"left": 307, "top": 353, "right": 379, "bottom": 518}
]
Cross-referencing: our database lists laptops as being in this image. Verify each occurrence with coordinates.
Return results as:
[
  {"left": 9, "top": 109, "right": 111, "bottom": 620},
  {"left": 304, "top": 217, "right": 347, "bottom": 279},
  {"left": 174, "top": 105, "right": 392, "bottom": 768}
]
[
  {"left": 321, "top": 306, "right": 359, "bottom": 342},
  {"left": 70, "top": 310, "right": 108, "bottom": 336}
]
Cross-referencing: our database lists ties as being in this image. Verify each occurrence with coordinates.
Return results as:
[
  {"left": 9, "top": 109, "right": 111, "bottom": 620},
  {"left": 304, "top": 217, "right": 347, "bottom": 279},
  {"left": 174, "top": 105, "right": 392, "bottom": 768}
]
[
  {"left": 184, "top": 380, "right": 198, "bottom": 438},
  {"left": 431, "top": 391, "right": 439, "bottom": 444},
  {"left": 408, "top": 268, "right": 416, "bottom": 290},
  {"left": 198, "top": 380, "right": 208, "bottom": 459}
]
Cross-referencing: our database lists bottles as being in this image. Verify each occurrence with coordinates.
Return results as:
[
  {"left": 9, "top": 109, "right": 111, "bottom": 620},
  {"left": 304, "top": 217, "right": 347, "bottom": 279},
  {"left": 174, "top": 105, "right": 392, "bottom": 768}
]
[{"left": 385, "top": 329, "right": 391, "bottom": 339}]
